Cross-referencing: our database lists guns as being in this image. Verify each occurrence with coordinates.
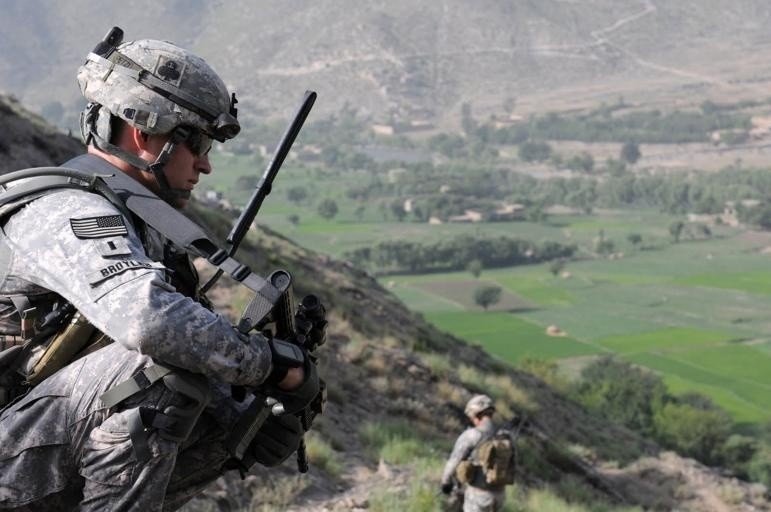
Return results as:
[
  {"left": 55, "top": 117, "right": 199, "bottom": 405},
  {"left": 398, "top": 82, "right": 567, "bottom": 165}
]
[
  {"left": 237, "top": 269, "right": 327, "bottom": 474},
  {"left": 433, "top": 415, "right": 525, "bottom": 497}
]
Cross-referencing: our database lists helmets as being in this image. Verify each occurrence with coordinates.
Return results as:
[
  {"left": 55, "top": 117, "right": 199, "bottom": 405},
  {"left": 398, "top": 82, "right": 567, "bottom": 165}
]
[
  {"left": 75, "top": 41, "right": 240, "bottom": 157},
  {"left": 465, "top": 394, "right": 491, "bottom": 418}
]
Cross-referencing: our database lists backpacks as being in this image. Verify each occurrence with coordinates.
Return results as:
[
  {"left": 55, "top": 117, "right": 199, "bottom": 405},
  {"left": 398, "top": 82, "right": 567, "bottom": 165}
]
[{"left": 478, "top": 427, "right": 516, "bottom": 488}]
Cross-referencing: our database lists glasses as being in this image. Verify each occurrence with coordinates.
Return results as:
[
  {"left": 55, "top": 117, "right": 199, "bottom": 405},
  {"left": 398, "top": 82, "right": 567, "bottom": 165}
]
[{"left": 172, "top": 124, "right": 214, "bottom": 159}]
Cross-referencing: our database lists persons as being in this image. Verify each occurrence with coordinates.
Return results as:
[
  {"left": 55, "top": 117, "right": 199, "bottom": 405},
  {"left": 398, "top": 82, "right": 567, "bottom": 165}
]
[
  {"left": 0, "top": 37, "right": 319, "bottom": 512},
  {"left": 442, "top": 395, "right": 506, "bottom": 512}
]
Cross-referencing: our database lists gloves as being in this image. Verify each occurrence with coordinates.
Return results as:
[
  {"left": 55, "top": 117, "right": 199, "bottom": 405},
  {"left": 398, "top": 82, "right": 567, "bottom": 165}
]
[{"left": 251, "top": 354, "right": 321, "bottom": 467}]
